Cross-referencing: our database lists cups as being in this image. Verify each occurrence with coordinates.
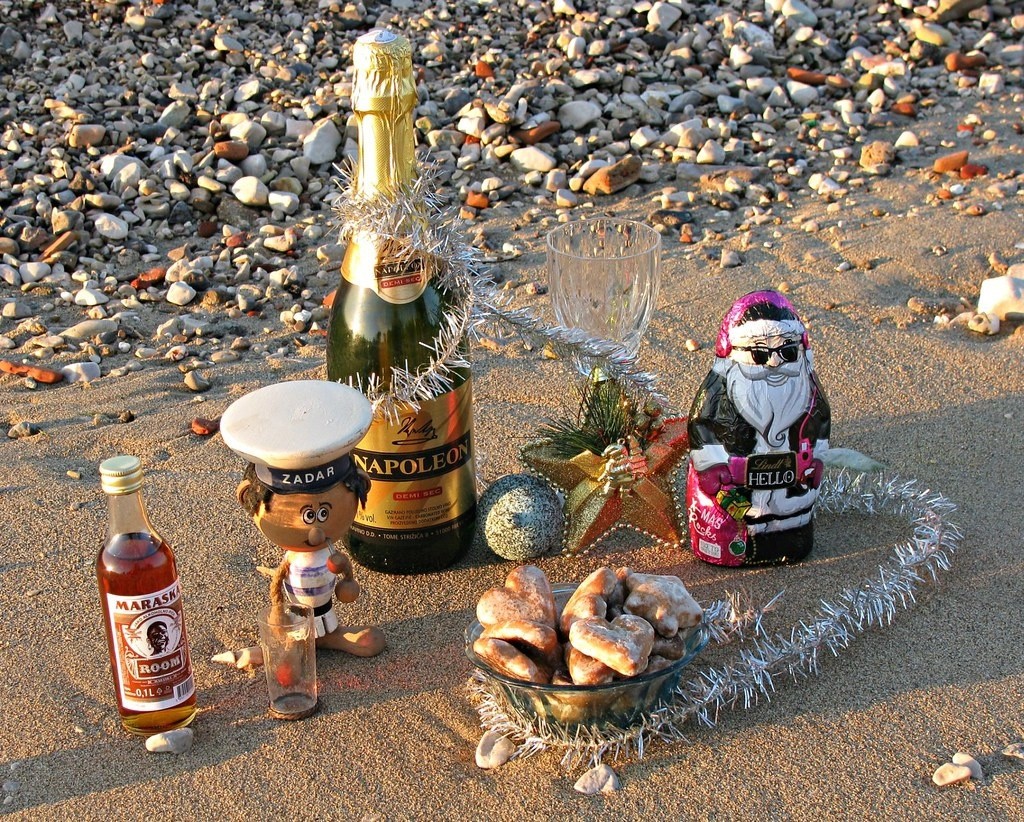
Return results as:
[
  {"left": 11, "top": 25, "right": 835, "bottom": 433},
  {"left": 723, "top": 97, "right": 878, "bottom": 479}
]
[
  {"left": 258, "top": 603, "right": 317, "bottom": 714},
  {"left": 547, "top": 216, "right": 662, "bottom": 381}
]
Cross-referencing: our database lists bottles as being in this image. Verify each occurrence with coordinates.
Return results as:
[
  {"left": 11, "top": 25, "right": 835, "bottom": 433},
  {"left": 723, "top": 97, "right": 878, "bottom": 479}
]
[
  {"left": 97, "top": 454, "right": 198, "bottom": 736},
  {"left": 326, "top": 30, "right": 475, "bottom": 575}
]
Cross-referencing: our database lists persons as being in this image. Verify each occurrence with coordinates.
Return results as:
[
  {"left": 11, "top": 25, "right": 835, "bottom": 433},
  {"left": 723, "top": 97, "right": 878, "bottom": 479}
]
[
  {"left": 687, "top": 290, "right": 831, "bottom": 567},
  {"left": 221, "top": 380, "right": 386, "bottom": 686}
]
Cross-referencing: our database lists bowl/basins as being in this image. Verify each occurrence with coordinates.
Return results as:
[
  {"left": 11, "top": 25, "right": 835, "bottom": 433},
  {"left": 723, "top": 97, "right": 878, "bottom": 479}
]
[{"left": 464, "top": 584, "right": 711, "bottom": 742}]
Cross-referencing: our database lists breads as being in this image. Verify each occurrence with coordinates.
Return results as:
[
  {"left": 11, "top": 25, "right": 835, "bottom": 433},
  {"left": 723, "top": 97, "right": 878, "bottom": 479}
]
[{"left": 478, "top": 565, "right": 704, "bottom": 726}]
